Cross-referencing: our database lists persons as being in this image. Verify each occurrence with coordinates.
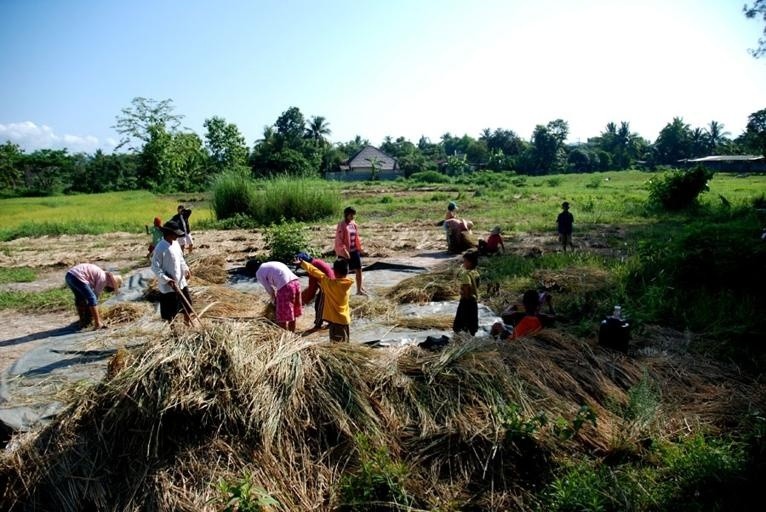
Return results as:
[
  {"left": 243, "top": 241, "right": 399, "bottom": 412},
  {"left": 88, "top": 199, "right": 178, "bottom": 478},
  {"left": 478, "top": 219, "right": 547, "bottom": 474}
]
[
  {"left": 144, "top": 204, "right": 195, "bottom": 337},
  {"left": 65, "top": 262, "right": 122, "bottom": 330},
  {"left": 444, "top": 201, "right": 558, "bottom": 338},
  {"left": 556, "top": 201, "right": 576, "bottom": 253},
  {"left": 247, "top": 206, "right": 369, "bottom": 345}
]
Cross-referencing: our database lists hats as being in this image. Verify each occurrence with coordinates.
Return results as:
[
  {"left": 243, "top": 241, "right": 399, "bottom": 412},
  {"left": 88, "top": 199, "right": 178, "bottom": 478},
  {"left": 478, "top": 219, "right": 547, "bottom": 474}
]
[
  {"left": 450, "top": 202, "right": 458, "bottom": 209},
  {"left": 296, "top": 253, "right": 311, "bottom": 267},
  {"left": 159, "top": 220, "right": 185, "bottom": 238},
  {"left": 108, "top": 271, "right": 122, "bottom": 292},
  {"left": 462, "top": 218, "right": 474, "bottom": 235},
  {"left": 155, "top": 216, "right": 161, "bottom": 226}
]
